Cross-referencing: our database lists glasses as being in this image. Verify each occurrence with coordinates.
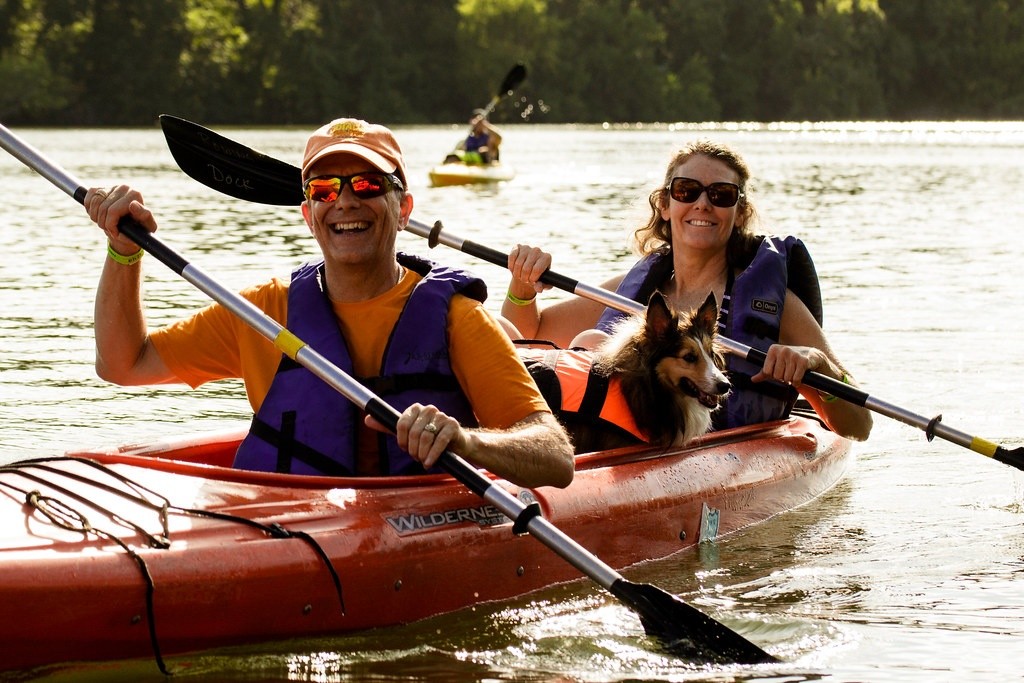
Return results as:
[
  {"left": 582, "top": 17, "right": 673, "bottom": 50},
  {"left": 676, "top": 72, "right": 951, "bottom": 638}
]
[
  {"left": 666, "top": 176, "right": 744, "bottom": 208},
  {"left": 302, "top": 168, "right": 405, "bottom": 204}
]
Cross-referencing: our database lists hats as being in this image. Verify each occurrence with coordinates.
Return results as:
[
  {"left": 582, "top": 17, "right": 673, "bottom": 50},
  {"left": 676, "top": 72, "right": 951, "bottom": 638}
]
[{"left": 300, "top": 117, "right": 408, "bottom": 191}]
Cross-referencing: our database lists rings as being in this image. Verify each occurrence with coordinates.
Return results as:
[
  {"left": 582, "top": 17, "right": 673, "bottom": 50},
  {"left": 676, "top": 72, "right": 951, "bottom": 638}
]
[
  {"left": 94, "top": 189, "right": 109, "bottom": 199},
  {"left": 423, "top": 422, "right": 438, "bottom": 436}
]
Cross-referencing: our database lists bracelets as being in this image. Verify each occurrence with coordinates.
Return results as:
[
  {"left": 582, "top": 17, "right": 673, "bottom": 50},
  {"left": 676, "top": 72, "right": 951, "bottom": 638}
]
[
  {"left": 506, "top": 287, "right": 538, "bottom": 306},
  {"left": 106, "top": 239, "right": 145, "bottom": 266},
  {"left": 816, "top": 371, "right": 853, "bottom": 403}
]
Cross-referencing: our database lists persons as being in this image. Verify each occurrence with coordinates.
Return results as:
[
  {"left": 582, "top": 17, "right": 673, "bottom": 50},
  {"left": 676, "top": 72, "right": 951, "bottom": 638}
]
[
  {"left": 500, "top": 143, "right": 874, "bottom": 444},
  {"left": 455, "top": 109, "right": 501, "bottom": 165},
  {"left": 83, "top": 117, "right": 575, "bottom": 491}
]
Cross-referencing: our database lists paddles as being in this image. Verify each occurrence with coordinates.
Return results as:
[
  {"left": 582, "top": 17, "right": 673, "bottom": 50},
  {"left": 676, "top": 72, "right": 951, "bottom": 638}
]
[
  {"left": 156, "top": 111, "right": 1024, "bottom": 472},
  {"left": 0, "top": 120, "right": 781, "bottom": 663},
  {"left": 457, "top": 61, "right": 529, "bottom": 150}
]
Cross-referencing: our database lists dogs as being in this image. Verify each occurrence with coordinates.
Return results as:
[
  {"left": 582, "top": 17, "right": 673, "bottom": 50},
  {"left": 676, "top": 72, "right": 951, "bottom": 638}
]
[{"left": 512, "top": 290, "right": 732, "bottom": 454}]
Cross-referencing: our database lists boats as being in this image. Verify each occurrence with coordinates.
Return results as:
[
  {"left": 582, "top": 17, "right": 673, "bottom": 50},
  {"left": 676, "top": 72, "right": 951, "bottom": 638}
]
[
  {"left": 0, "top": 392, "right": 861, "bottom": 682},
  {"left": 426, "top": 159, "right": 517, "bottom": 187}
]
